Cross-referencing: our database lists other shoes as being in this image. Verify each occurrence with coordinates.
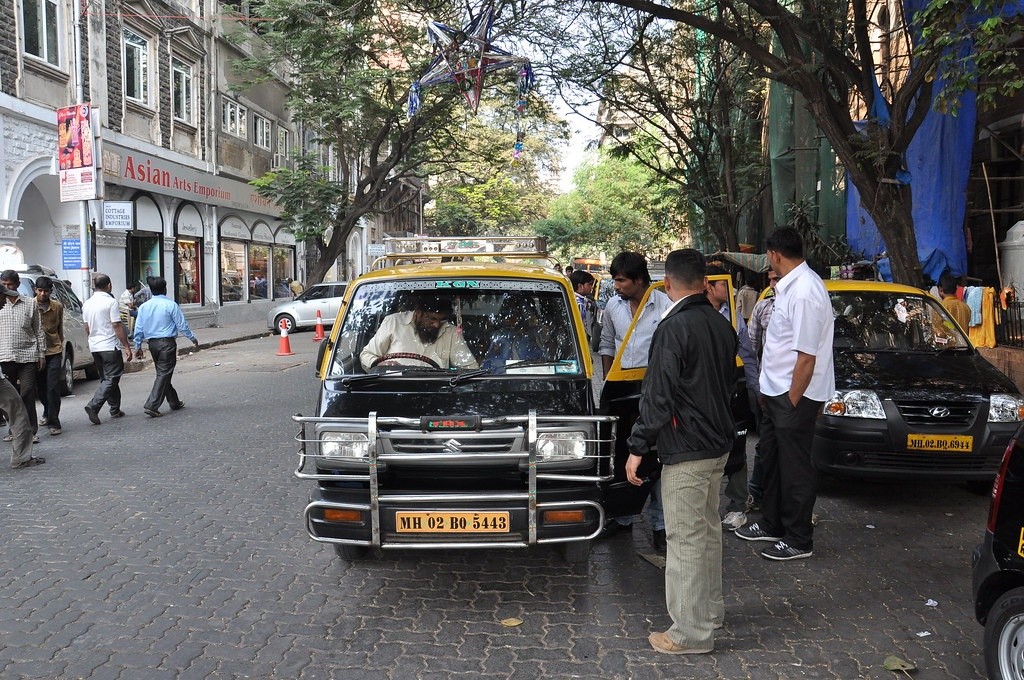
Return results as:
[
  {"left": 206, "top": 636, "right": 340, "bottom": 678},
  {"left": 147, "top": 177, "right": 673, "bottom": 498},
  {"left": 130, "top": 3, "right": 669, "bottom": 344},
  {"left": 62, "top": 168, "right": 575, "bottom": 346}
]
[
  {"left": 3, "top": 435, "right": 12, "bottom": 441},
  {"left": 50, "top": 428, "right": 62, "bottom": 435},
  {"left": 143, "top": 408, "right": 163, "bottom": 417},
  {"left": 653, "top": 530, "right": 667, "bottom": 551},
  {"left": 13, "top": 456, "right": 46, "bottom": 469},
  {"left": 32, "top": 435, "right": 40, "bottom": 443},
  {"left": 598, "top": 519, "right": 633, "bottom": 535},
  {"left": 38, "top": 417, "right": 47, "bottom": 426},
  {"left": 172, "top": 401, "right": 185, "bottom": 410},
  {"left": 110, "top": 411, "right": 125, "bottom": 420},
  {"left": 85, "top": 405, "right": 101, "bottom": 424}
]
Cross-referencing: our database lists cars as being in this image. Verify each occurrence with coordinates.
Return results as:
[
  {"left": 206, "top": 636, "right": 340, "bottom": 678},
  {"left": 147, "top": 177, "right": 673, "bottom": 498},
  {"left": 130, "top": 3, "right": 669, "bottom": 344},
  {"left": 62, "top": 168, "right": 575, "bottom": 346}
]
[
  {"left": 751, "top": 280, "right": 1023, "bottom": 475},
  {"left": 266, "top": 282, "right": 356, "bottom": 333},
  {"left": 0, "top": 264, "right": 104, "bottom": 395},
  {"left": 971, "top": 414, "right": 1023, "bottom": 680},
  {"left": 289, "top": 264, "right": 748, "bottom": 561}
]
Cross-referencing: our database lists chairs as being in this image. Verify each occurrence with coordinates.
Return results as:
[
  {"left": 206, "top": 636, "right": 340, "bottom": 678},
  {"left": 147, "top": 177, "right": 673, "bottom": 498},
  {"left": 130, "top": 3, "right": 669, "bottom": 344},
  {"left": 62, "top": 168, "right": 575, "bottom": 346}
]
[
  {"left": 834, "top": 312, "right": 913, "bottom": 347},
  {"left": 397, "top": 297, "right": 560, "bottom": 364},
  {"left": 119, "top": 306, "right": 138, "bottom": 334}
]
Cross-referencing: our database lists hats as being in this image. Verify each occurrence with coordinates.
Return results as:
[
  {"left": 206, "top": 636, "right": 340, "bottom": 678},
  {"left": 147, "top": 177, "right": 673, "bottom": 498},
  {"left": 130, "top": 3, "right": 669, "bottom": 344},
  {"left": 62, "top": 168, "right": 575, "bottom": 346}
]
[{"left": 0, "top": 284, "right": 20, "bottom": 296}]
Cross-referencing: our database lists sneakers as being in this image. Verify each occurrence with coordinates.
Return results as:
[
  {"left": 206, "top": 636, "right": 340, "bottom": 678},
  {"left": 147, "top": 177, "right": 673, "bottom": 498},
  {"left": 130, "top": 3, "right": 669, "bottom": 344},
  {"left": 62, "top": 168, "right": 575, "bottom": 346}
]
[
  {"left": 726, "top": 495, "right": 760, "bottom": 514},
  {"left": 735, "top": 522, "right": 783, "bottom": 541},
  {"left": 722, "top": 512, "right": 748, "bottom": 530},
  {"left": 649, "top": 632, "right": 714, "bottom": 654},
  {"left": 761, "top": 541, "right": 813, "bottom": 560}
]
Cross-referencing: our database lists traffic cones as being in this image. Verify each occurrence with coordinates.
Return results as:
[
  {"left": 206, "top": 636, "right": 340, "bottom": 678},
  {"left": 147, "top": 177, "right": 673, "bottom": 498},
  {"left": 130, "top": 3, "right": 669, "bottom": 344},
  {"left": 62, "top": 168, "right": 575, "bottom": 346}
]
[
  {"left": 275, "top": 320, "right": 296, "bottom": 355},
  {"left": 310, "top": 310, "right": 327, "bottom": 341}
]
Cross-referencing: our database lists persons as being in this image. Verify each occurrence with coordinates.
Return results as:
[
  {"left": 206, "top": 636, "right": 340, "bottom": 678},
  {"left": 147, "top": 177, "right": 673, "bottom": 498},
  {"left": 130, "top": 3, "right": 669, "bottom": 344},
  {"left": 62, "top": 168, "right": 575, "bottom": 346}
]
[
  {"left": 626, "top": 248, "right": 737, "bottom": 654},
  {"left": 601, "top": 251, "right": 669, "bottom": 550},
  {"left": 929, "top": 276, "right": 971, "bottom": 345},
  {"left": 0, "top": 270, "right": 47, "bottom": 443},
  {"left": 32, "top": 276, "right": 65, "bottom": 434},
  {"left": 0, "top": 283, "right": 46, "bottom": 469},
  {"left": 359, "top": 297, "right": 479, "bottom": 374},
  {"left": 120, "top": 276, "right": 154, "bottom": 338},
  {"left": 705, "top": 227, "right": 783, "bottom": 529},
  {"left": 736, "top": 224, "right": 837, "bottom": 560},
  {"left": 554, "top": 264, "right": 595, "bottom": 363},
  {"left": 81, "top": 273, "right": 133, "bottom": 424},
  {"left": 63, "top": 279, "right": 72, "bottom": 287},
  {"left": 133, "top": 277, "right": 199, "bottom": 417}
]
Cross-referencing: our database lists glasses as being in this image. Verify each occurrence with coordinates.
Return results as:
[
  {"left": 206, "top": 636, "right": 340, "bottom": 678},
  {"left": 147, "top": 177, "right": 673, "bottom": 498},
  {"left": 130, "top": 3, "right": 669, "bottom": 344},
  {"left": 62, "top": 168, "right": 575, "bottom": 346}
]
[{"left": 422, "top": 311, "right": 450, "bottom": 324}]
